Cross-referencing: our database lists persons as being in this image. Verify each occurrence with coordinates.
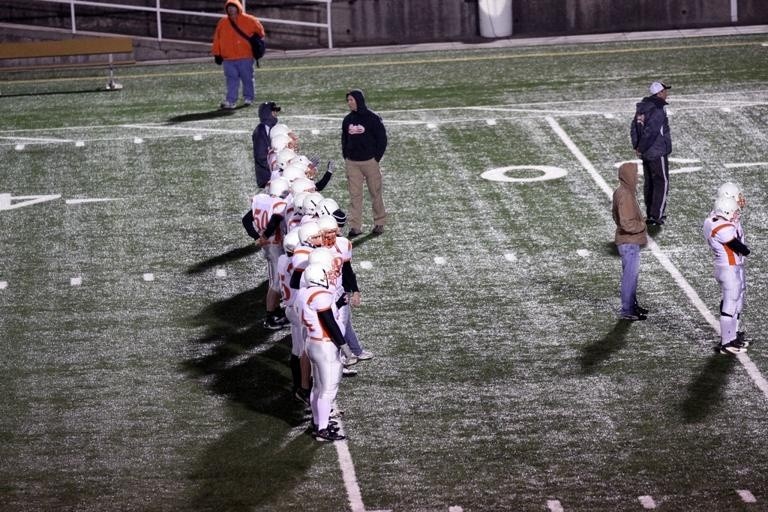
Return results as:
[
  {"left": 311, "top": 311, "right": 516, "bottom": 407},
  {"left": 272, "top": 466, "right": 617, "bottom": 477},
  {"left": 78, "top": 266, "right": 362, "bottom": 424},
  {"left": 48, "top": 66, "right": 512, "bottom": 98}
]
[
  {"left": 627, "top": 81, "right": 673, "bottom": 226},
  {"left": 341, "top": 91, "right": 387, "bottom": 236},
  {"left": 210, "top": 0, "right": 270, "bottom": 111},
  {"left": 700, "top": 198, "right": 751, "bottom": 356},
  {"left": 244, "top": 100, "right": 373, "bottom": 441},
  {"left": 720, "top": 181, "right": 752, "bottom": 347},
  {"left": 610, "top": 163, "right": 649, "bottom": 321}
]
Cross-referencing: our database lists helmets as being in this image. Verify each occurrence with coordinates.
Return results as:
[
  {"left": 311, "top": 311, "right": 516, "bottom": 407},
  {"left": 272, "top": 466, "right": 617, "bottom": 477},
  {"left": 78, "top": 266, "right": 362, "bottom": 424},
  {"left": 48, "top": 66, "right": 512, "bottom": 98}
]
[
  {"left": 294, "top": 192, "right": 311, "bottom": 216},
  {"left": 308, "top": 248, "right": 333, "bottom": 273},
  {"left": 276, "top": 148, "right": 297, "bottom": 172},
  {"left": 713, "top": 199, "right": 741, "bottom": 222},
  {"left": 315, "top": 198, "right": 340, "bottom": 219},
  {"left": 302, "top": 193, "right": 324, "bottom": 215},
  {"left": 316, "top": 215, "right": 338, "bottom": 233},
  {"left": 283, "top": 230, "right": 302, "bottom": 253},
  {"left": 717, "top": 181, "right": 742, "bottom": 202},
  {"left": 270, "top": 177, "right": 291, "bottom": 197},
  {"left": 272, "top": 134, "right": 291, "bottom": 153},
  {"left": 282, "top": 165, "right": 305, "bottom": 179},
  {"left": 298, "top": 221, "right": 322, "bottom": 248},
  {"left": 291, "top": 178, "right": 316, "bottom": 199},
  {"left": 299, "top": 215, "right": 318, "bottom": 224},
  {"left": 290, "top": 156, "right": 312, "bottom": 171},
  {"left": 291, "top": 246, "right": 314, "bottom": 270},
  {"left": 270, "top": 125, "right": 292, "bottom": 140},
  {"left": 304, "top": 264, "right": 329, "bottom": 289}
]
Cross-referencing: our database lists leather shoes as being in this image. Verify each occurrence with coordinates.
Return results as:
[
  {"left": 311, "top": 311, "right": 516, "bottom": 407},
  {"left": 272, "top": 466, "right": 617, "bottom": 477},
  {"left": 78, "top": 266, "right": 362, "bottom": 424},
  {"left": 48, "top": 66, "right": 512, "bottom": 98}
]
[
  {"left": 661, "top": 215, "right": 668, "bottom": 219},
  {"left": 646, "top": 218, "right": 664, "bottom": 225}
]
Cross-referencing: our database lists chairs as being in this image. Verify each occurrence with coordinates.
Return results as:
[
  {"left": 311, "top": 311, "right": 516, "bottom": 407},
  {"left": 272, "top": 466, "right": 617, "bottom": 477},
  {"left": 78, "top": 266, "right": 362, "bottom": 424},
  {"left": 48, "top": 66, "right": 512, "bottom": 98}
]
[{"left": 0, "top": 36, "right": 134, "bottom": 92}]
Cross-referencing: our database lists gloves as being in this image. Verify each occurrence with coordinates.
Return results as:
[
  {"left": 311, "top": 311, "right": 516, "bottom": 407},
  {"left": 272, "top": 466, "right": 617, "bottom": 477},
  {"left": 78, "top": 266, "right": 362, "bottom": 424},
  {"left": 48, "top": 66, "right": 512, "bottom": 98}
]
[
  {"left": 327, "top": 160, "right": 337, "bottom": 174},
  {"left": 308, "top": 152, "right": 320, "bottom": 171},
  {"left": 341, "top": 344, "right": 352, "bottom": 363},
  {"left": 214, "top": 55, "right": 224, "bottom": 65}
]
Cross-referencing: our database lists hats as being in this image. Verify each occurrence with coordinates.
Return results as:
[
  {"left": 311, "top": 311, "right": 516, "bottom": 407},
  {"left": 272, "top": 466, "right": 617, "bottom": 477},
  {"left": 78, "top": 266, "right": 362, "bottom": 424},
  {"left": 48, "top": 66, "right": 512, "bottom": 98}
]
[
  {"left": 649, "top": 82, "right": 672, "bottom": 95},
  {"left": 272, "top": 105, "right": 281, "bottom": 112}
]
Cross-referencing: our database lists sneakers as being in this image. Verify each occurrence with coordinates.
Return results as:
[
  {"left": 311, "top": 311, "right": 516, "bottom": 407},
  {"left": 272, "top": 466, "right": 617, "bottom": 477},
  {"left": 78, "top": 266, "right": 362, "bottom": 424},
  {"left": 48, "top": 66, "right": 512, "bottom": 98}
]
[
  {"left": 221, "top": 101, "right": 237, "bottom": 109},
  {"left": 720, "top": 342, "right": 748, "bottom": 354},
  {"left": 264, "top": 320, "right": 282, "bottom": 330},
  {"left": 620, "top": 312, "right": 647, "bottom": 321},
  {"left": 274, "top": 316, "right": 292, "bottom": 328},
  {"left": 636, "top": 307, "right": 649, "bottom": 315},
  {"left": 295, "top": 388, "right": 310, "bottom": 406},
  {"left": 343, "top": 368, "right": 357, "bottom": 377},
  {"left": 349, "top": 228, "right": 363, "bottom": 237},
  {"left": 344, "top": 359, "right": 357, "bottom": 365},
  {"left": 312, "top": 424, "right": 346, "bottom": 441},
  {"left": 372, "top": 225, "right": 384, "bottom": 236},
  {"left": 731, "top": 339, "right": 749, "bottom": 347},
  {"left": 351, "top": 350, "right": 373, "bottom": 359},
  {"left": 244, "top": 99, "right": 253, "bottom": 105}
]
